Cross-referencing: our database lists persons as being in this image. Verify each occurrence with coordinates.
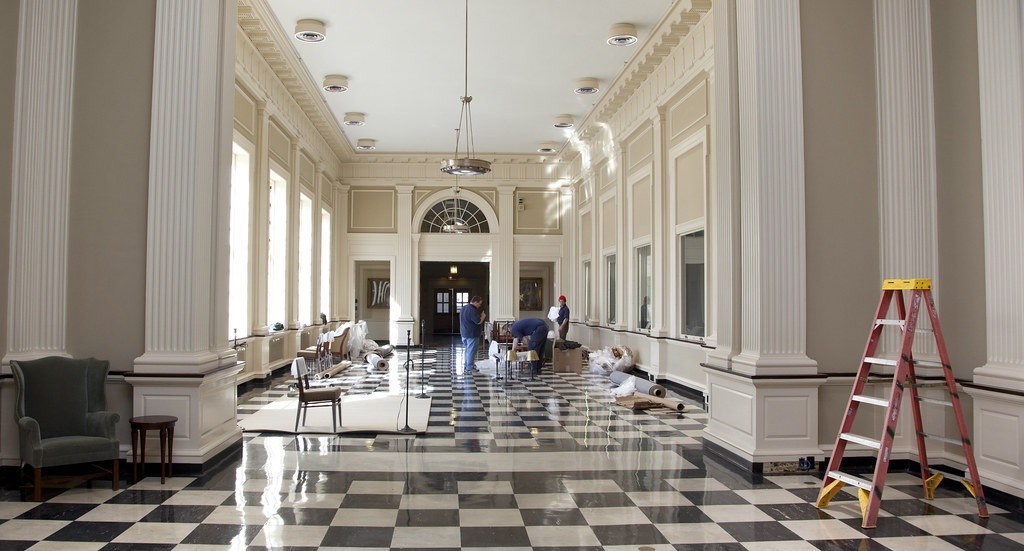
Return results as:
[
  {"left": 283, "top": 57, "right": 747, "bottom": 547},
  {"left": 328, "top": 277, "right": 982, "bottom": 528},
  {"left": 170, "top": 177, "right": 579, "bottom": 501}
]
[
  {"left": 558, "top": 295, "right": 570, "bottom": 340},
  {"left": 460, "top": 296, "right": 486, "bottom": 372},
  {"left": 502, "top": 318, "right": 549, "bottom": 375},
  {"left": 641, "top": 296, "right": 650, "bottom": 328}
]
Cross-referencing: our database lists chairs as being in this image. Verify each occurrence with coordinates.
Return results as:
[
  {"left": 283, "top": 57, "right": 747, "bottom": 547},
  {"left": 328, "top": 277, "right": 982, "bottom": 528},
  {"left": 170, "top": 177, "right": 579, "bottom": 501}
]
[
  {"left": 297, "top": 330, "right": 334, "bottom": 373},
  {"left": 9, "top": 356, "right": 121, "bottom": 502},
  {"left": 295, "top": 357, "right": 342, "bottom": 432}
]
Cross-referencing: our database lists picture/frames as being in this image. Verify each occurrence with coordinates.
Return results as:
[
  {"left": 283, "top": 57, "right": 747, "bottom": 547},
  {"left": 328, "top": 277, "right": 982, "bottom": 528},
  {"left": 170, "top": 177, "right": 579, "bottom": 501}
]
[
  {"left": 519, "top": 276, "right": 543, "bottom": 311},
  {"left": 367, "top": 277, "right": 390, "bottom": 308}
]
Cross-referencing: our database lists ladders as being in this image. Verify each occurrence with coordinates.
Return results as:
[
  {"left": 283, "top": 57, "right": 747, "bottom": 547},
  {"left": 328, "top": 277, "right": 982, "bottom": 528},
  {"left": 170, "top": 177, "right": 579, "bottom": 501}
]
[{"left": 816, "top": 278, "right": 990, "bottom": 529}]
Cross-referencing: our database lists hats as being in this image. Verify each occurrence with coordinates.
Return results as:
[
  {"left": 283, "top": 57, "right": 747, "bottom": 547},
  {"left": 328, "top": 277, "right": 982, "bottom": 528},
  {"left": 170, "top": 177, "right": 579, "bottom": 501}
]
[{"left": 558, "top": 295, "right": 566, "bottom": 301}]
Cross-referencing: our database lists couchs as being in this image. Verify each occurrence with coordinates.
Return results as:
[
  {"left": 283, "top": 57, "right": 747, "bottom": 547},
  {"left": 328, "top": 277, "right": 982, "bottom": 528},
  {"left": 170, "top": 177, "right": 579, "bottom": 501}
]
[{"left": 331, "top": 326, "right": 351, "bottom": 362}]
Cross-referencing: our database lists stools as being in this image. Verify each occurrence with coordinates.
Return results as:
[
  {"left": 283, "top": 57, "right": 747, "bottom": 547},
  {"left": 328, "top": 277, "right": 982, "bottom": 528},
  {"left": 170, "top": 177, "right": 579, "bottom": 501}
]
[{"left": 129, "top": 414, "right": 178, "bottom": 484}]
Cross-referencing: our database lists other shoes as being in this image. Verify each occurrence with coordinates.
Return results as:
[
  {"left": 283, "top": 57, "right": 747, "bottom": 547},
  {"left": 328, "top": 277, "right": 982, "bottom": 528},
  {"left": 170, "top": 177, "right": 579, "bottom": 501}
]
[
  {"left": 463, "top": 369, "right": 481, "bottom": 374},
  {"left": 525, "top": 368, "right": 542, "bottom": 375}
]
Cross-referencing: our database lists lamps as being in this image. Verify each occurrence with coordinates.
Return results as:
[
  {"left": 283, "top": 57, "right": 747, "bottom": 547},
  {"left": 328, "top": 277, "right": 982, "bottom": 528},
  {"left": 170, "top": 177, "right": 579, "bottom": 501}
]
[
  {"left": 356, "top": 138, "right": 375, "bottom": 151},
  {"left": 606, "top": 23, "right": 639, "bottom": 46},
  {"left": 574, "top": 76, "right": 599, "bottom": 95},
  {"left": 323, "top": 74, "right": 349, "bottom": 93},
  {"left": 442, "top": 128, "right": 469, "bottom": 232},
  {"left": 440, "top": 0, "right": 491, "bottom": 177},
  {"left": 294, "top": 18, "right": 326, "bottom": 43},
  {"left": 553, "top": 114, "right": 574, "bottom": 127},
  {"left": 344, "top": 111, "right": 366, "bottom": 127},
  {"left": 536, "top": 143, "right": 557, "bottom": 152}
]
